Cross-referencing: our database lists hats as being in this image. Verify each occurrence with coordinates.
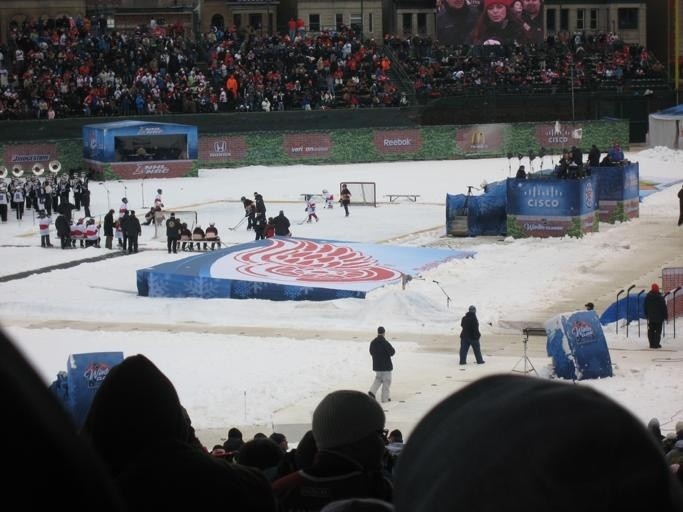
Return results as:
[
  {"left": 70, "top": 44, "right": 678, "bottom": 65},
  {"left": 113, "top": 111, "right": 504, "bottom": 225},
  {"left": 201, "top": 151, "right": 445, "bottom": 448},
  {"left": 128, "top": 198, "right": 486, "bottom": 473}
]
[
  {"left": 585, "top": 303, "right": 594, "bottom": 309},
  {"left": 469, "top": 305, "right": 476, "bottom": 312},
  {"left": 310, "top": 389, "right": 385, "bottom": 453},
  {"left": 378, "top": 327, "right": 386, "bottom": 334},
  {"left": 652, "top": 283, "right": 660, "bottom": 292}
]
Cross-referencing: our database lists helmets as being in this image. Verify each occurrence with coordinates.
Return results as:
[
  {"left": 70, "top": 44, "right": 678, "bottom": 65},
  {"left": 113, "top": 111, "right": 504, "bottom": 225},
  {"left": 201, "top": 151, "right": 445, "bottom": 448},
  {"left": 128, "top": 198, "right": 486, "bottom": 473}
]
[
  {"left": 209, "top": 221, "right": 215, "bottom": 226},
  {"left": 342, "top": 184, "right": 347, "bottom": 188},
  {"left": 78, "top": 218, "right": 94, "bottom": 224},
  {"left": 306, "top": 194, "right": 311, "bottom": 200},
  {"left": 183, "top": 222, "right": 188, "bottom": 227},
  {"left": 196, "top": 223, "right": 200, "bottom": 228},
  {"left": 322, "top": 188, "right": 327, "bottom": 192}
]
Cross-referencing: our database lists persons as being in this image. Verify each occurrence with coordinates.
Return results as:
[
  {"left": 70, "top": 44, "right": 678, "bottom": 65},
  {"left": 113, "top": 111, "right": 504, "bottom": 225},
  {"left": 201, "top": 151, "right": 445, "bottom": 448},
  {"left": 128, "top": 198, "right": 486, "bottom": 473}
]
[
  {"left": 141, "top": 189, "right": 164, "bottom": 225},
  {"left": 459, "top": 305, "right": 485, "bottom": 364},
  {"left": 2, "top": 14, "right": 660, "bottom": 120},
  {"left": 322, "top": 189, "right": 334, "bottom": 208},
  {"left": 1, "top": 330, "right": 683, "bottom": 512},
  {"left": 136, "top": 145, "right": 162, "bottom": 160},
  {"left": 2, "top": 171, "right": 91, "bottom": 221},
  {"left": 36, "top": 200, "right": 101, "bottom": 248},
  {"left": 644, "top": 284, "right": 669, "bottom": 348},
  {"left": 305, "top": 194, "right": 319, "bottom": 223},
  {"left": 516, "top": 166, "right": 527, "bottom": 178},
  {"left": 104, "top": 198, "right": 142, "bottom": 253},
  {"left": 166, "top": 213, "right": 221, "bottom": 253},
  {"left": 586, "top": 302, "right": 594, "bottom": 311},
  {"left": 562, "top": 144, "right": 612, "bottom": 168},
  {"left": 241, "top": 192, "right": 289, "bottom": 240},
  {"left": 339, "top": 184, "right": 352, "bottom": 216},
  {"left": 368, "top": 326, "right": 395, "bottom": 402},
  {"left": 436, "top": 2, "right": 540, "bottom": 45}
]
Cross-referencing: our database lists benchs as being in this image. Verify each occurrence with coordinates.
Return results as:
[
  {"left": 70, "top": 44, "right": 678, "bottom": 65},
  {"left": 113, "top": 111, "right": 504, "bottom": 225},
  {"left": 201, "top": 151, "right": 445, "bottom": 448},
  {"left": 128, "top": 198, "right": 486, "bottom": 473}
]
[
  {"left": 0, "top": 15, "right": 220, "bottom": 117},
  {"left": 384, "top": 36, "right": 592, "bottom": 101},
  {"left": 543, "top": 34, "right": 671, "bottom": 93},
  {"left": 298, "top": 193, "right": 323, "bottom": 202},
  {"left": 383, "top": 193, "right": 422, "bottom": 202},
  {"left": 175, "top": 238, "right": 223, "bottom": 253},
  {"left": 53, "top": 235, "right": 100, "bottom": 249},
  {"left": 194, "top": 28, "right": 414, "bottom": 112}
]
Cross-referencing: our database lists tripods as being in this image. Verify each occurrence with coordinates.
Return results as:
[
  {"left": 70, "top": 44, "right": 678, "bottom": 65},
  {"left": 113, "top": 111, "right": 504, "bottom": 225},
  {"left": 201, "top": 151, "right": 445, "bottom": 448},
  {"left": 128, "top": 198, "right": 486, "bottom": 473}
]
[{"left": 511, "top": 344, "right": 540, "bottom": 377}]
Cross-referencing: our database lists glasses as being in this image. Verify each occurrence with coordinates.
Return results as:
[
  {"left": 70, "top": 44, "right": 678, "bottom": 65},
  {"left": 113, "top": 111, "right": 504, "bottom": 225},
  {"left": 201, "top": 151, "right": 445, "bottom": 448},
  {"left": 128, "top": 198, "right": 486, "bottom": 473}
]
[{"left": 382, "top": 429, "right": 388, "bottom": 437}]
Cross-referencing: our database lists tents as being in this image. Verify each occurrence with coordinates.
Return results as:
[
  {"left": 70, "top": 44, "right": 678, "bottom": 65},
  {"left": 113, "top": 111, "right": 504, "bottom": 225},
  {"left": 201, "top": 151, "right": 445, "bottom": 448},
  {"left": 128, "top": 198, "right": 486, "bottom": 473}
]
[{"left": 80, "top": 120, "right": 199, "bottom": 162}]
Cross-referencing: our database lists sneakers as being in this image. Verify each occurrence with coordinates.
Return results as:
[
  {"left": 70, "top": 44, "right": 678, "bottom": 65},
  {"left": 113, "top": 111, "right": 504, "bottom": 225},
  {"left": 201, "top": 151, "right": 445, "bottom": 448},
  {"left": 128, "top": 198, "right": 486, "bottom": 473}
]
[
  {"left": 382, "top": 398, "right": 391, "bottom": 404},
  {"left": 460, "top": 362, "right": 467, "bottom": 365},
  {"left": 477, "top": 361, "right": 485, "bottom": 364},
  {"left": 368, "top": 391, "right": 376, "bottom": 399},
  {"left": 649, "top": 344, "right": 662, "bottom": 348}
]
